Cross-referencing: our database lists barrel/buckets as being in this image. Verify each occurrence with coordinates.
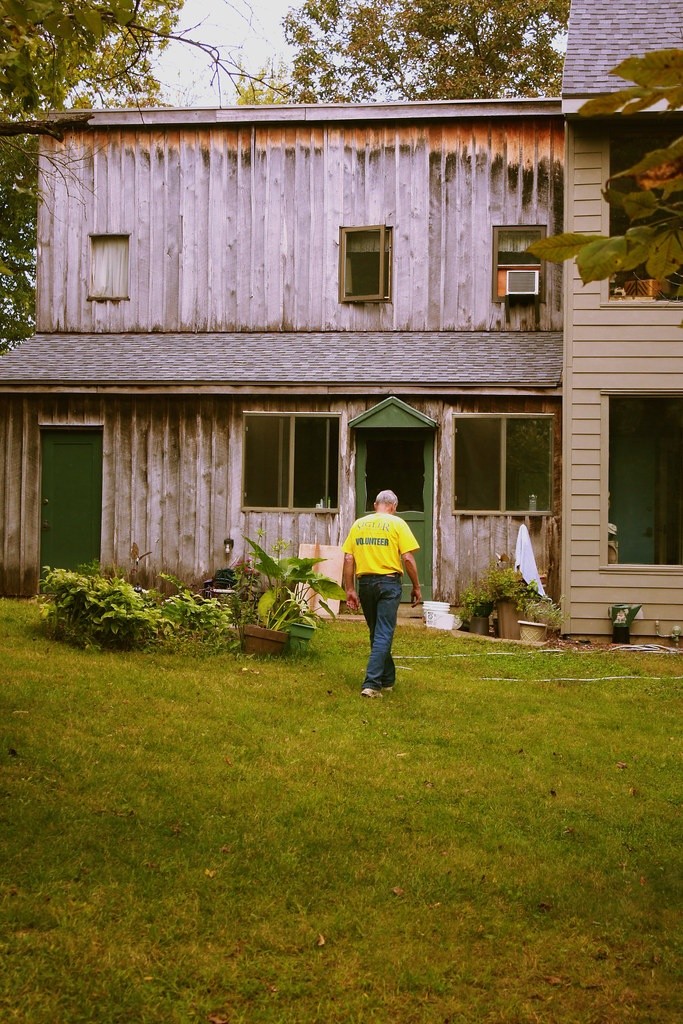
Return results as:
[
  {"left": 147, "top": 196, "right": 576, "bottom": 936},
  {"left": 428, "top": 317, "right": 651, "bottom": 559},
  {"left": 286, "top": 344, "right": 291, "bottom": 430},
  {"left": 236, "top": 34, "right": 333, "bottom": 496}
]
[
  {"left": 612, "top": 627, "right": 630, "bottom": 644},
  {"left": 423, "top": 600, "right": 455, "bottom": 631}
]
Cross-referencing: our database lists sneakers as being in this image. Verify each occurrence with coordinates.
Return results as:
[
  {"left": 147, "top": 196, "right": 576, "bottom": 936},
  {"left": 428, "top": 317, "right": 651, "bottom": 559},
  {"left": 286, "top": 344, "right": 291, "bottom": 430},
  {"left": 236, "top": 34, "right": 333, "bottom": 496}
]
[
  {"left": 361, "top": 688, "right": 382, "bottom": 698},
  {"left": 383, "top": 686, "right": 393, "bottom": 691}
]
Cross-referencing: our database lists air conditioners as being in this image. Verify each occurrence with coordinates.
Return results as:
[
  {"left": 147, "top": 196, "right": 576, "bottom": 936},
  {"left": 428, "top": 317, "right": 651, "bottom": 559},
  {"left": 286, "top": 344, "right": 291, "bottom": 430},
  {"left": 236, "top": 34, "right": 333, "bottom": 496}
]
[{"left": 506, "top": 270, "right": 539, "bottom": 296}]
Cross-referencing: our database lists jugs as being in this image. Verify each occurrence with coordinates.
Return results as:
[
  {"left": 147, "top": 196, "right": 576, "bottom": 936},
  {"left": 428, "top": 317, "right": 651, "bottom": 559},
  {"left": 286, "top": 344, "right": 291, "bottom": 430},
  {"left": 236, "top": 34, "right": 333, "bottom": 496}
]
[{"left": 607, "top": 604, "right": 642, "bottom": 627}]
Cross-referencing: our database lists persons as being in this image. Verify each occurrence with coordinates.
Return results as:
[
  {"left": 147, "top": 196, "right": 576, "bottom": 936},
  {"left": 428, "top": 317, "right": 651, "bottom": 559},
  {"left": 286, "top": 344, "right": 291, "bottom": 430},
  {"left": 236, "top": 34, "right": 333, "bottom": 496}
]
[{"left": 340, "top": 490, "right": 422, "bottom": 699}]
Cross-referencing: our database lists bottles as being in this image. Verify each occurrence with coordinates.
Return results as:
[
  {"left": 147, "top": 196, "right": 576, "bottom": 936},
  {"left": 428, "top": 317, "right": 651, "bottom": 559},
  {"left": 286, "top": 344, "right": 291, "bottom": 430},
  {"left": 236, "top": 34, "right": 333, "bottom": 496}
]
[
  {"left": 528, "top": 493, "right": 537, "bottom": 511},
  {"left": 315, "top": 496, "right": 331, "bottom": 508}
]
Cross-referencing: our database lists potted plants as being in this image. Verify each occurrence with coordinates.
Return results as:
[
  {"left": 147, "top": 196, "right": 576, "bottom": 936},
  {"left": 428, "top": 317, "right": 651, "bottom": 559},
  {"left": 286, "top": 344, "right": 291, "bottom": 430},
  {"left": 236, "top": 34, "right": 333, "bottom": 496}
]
[
  {"left": 458, "top": 557, "right": 571, "bottom": 644},
  {"left": 239, "top": 528, "right": 347, "bottom": 657}
]
